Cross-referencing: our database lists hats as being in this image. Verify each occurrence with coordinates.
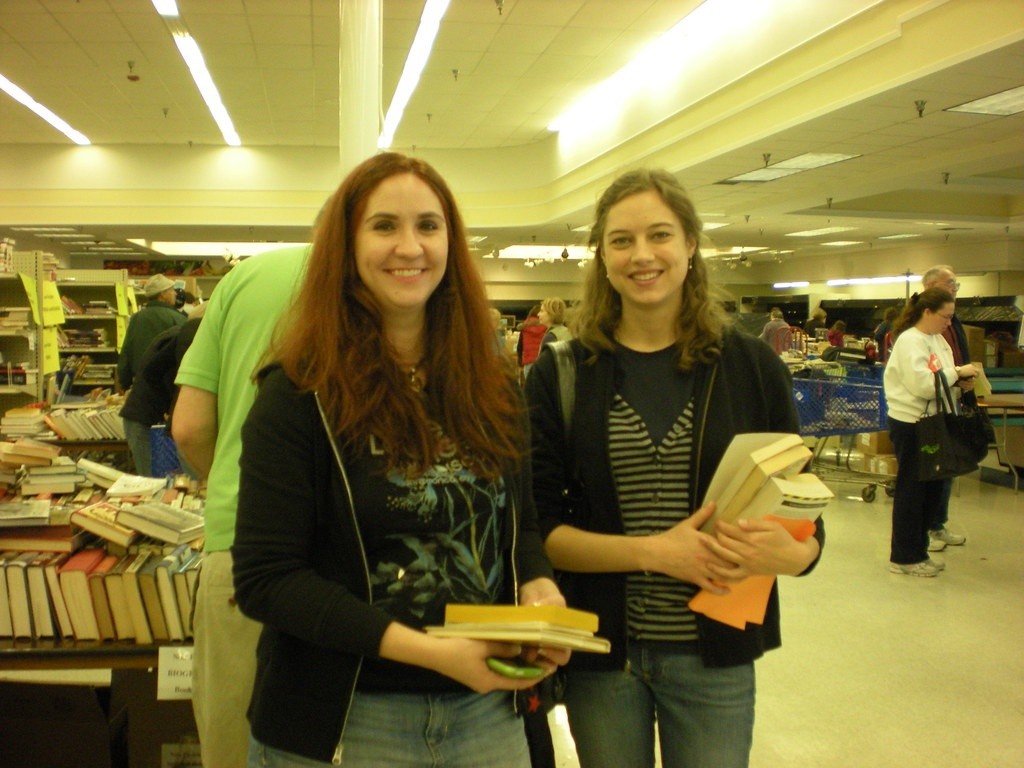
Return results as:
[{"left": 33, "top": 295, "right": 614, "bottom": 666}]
[{"left": 144, "top": 274, "right": 175, "bottom": 297}]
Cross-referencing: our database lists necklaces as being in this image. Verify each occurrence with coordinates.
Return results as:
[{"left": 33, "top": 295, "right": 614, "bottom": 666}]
[{"left": 407, "top": 358, "right": 426, "bottom": 390}]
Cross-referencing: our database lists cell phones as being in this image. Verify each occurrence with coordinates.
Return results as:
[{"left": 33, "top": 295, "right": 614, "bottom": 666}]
[{"left": 485, "top": 656, "right": 544, "bottom": 679}]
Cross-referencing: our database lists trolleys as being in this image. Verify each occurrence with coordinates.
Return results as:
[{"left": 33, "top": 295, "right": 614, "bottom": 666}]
[{"left": 789, "top": 367, "right": 897, "bottom": 504}]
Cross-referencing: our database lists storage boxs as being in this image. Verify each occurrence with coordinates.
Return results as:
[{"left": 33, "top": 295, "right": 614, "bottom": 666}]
[
  {"left": 863, "top": 454, "right": 898, "bottom": 476},
  {"left": 857, "top": 431, "right": 894, "bottom": 454},
  {"left": 963, "top": 325, "right": 1024, "bottom": 367}
]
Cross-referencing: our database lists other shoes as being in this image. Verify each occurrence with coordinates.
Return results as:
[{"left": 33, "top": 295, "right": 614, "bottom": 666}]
[
  {"left": 927, "top": 535, "right": 947, "bottom": 551},
  {"left": 890, "top": 551, "right": 945, "bottom": 577},
  {"left": 928, "top": 525, "right": 966, "bottom": 545}
]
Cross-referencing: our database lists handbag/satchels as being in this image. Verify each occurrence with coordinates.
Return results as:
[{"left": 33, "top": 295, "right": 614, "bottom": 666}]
[
  {"left": 151, "top": 426, "right": 183, "bottom": 478},
  {"left": 915, "top": 369, "right": 996, "bottom": 482}
]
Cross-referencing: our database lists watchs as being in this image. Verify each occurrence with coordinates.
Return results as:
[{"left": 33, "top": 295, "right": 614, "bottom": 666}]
[{"left": 953, "top": 365, "right": 962, "bottom": 378}]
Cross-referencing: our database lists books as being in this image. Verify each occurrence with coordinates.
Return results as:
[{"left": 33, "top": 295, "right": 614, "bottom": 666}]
[
  {"left": 970, "top": 361, "right": 993, "bottom": 401},
  {"left": 423, "top": 603, "right": 611, "bottom": 653},
  {"left": 686, "top": 432, "right": 835, "bottom": 537},
  {"left": 0, "top": 233, "right": 203, "bottom": 384},
  {"left": 0, "top": 386, "right": 210, "bottom": 645}
]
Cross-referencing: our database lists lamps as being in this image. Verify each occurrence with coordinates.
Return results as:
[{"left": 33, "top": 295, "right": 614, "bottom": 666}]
[
  {"left": 578, "top": 259, "right": 587, "bottom": 269},
  {"left": 727, "top": 256, "right": 736, "bottom": 270},
  {"left": 740, "top": 256, "right": 752, "bottom": 267},
  {"left": 561, "top": 248, "right": 568, "bottom": 262},
  {"left": 525, "top": 258, "right": 554, "bottom": 268}
]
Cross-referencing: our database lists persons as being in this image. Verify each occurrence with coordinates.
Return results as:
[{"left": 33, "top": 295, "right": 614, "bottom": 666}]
[
  {"left": 170, "top": 245, "right": 325, "bottom": 768},
  {"left": 118, "top": 273, "right": 212, "bottom": 478},
  {"left": 883, "top": 287, "right": 980, "bottom": 578},
  {"left": 231, "top": 153, "right": 571, "bottom": 768},
  {"left": 923, "top": 266, "right": 976, "bottom": 552},
  {"left": 523, "top": 168, "right": 825, "bottom": 768},
  {"left": 759, "top": 306, "right": 898, "bottom": 364},
  {"left": 485, "top": 298, "right": 573, "bottom": 380}
]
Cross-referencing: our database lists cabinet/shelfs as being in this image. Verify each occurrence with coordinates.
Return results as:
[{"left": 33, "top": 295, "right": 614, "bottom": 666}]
[
  {"left": 52, "top": 269, "right": 129, "bottom": 399},
  {"left": 0, "top": 250, "right": 43, "bottom": 416}
]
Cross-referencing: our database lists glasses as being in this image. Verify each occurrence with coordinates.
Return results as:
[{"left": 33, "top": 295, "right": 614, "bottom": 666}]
[{"left": 936, "top": 282, "right": 960, "bottom": 289}]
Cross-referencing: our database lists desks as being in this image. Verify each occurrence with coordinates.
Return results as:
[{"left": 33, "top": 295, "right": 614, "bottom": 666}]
[
  {"left": 43, "top": 439, "right": 131, "bottom": 473},
  {"left": 975, "top": 400, "right": 1024, "bottom": 493},
  {"left": 0, "top": 637, "right": 193, "bottom": 768}
]
